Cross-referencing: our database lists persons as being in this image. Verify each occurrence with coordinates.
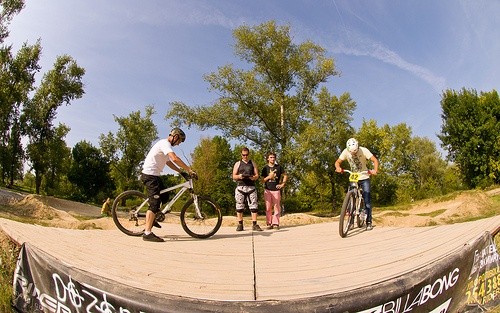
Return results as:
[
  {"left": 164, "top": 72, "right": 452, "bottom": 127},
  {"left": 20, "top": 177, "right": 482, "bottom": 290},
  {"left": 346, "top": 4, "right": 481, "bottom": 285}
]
[
  {"left": 140, "top": 128, "right": 192, "bottom": 242},
  {"left": 233, "top": 148, "right": 262, "bottom": 231},
  {"left": 260, "top": 153, "right": 287, "bottom": 228},
  {"left": 335, "top": 138, "right": 378, "bottom": 229}
]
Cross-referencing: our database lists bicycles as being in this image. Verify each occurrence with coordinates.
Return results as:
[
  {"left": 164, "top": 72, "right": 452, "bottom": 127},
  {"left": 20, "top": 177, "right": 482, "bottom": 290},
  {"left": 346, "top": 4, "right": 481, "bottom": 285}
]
[
  {"left": 335, "top": 169, "right": 370, "bottom": 237},
  {"left": 112, "top": 175, "right": 222, "bottom": 239}
]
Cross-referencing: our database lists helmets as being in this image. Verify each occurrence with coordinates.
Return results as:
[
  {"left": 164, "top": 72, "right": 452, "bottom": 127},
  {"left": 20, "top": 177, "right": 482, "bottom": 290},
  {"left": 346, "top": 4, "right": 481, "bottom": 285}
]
[
  {"left": 346, "top": 138, "right": 358, "bottom": 155},
  {"left": 169, "top": 128, "right": 186, "bottom": 142}
]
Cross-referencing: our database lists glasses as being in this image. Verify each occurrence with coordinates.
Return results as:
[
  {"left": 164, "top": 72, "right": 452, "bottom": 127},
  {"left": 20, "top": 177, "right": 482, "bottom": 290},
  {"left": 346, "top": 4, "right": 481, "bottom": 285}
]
[{"left": 242, "top": 154, "right": 249, "bottom": 156}]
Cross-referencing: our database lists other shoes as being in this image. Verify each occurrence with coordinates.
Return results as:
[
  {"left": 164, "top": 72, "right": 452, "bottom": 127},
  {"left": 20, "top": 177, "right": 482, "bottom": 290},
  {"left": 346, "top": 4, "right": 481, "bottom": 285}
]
[
  {"left": 253, "top": 225, "right": 263, "bottom": 231},
  {"left": 366, "top": 222, "right": 372, "bottom": 230},
  {"left": 236, "top": 224, "right": 244, "bottom": 231},
  {"left": 273, "top": 224, "right": 278, "bottom": 229},
  {"left": 152, "top": 220, "right": 160, "bottom": 228},
  {"left": 143, "top": 233, "right": 163, "bottom": 242},
  {"left": 266, "top": 224, "right": 271, "bottom": 229}
]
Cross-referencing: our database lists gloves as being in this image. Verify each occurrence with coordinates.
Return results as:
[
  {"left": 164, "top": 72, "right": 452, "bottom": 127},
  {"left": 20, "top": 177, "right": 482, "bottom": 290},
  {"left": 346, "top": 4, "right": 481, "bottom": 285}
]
[
  {"left": 189, "top": 171, "right": 199, "bottom": 180},
  {"left": 182, "top": 173, "right": 190, "bottom": 180}
]
